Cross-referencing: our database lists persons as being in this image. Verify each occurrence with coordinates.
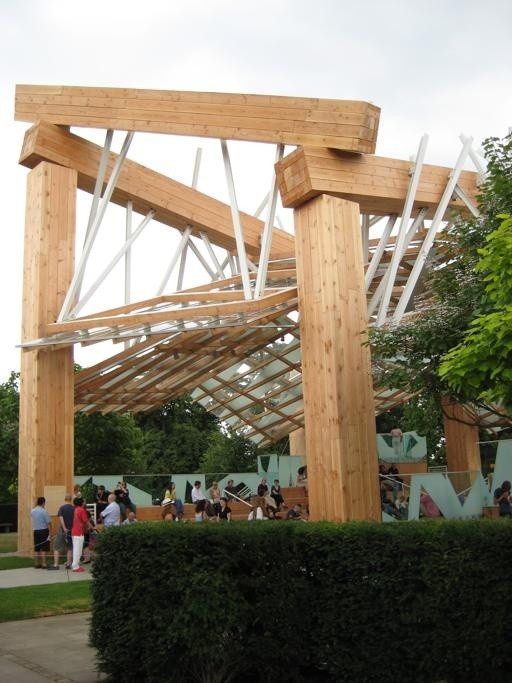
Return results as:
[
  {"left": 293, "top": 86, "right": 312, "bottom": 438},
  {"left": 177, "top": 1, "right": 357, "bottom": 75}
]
[
  {"left": 389, "top": 424, "right": 404, "bottom": 455},
  {"left": 493, "top": 480, "right": 512, "bottom": 516},
  {"left": 29, "top": 464, "right": 309, "bottom": 572},
  {"left": 378, "top": 462, "right": 441, "bottom": 518}
]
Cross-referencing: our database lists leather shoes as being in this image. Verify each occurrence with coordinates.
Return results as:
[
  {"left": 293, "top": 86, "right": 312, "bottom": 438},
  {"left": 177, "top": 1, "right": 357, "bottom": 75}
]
[{"left": 83, "top": 560, "right": 91, "bottom": 564}]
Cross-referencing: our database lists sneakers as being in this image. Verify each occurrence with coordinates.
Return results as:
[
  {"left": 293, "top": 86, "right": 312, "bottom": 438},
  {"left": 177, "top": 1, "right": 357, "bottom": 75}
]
[
  {"left": 66, "top": 563, "right": 83, "bottom": 572},
  {"left": 35, "top": 563, "right": 59, "bottom": 570}
]
